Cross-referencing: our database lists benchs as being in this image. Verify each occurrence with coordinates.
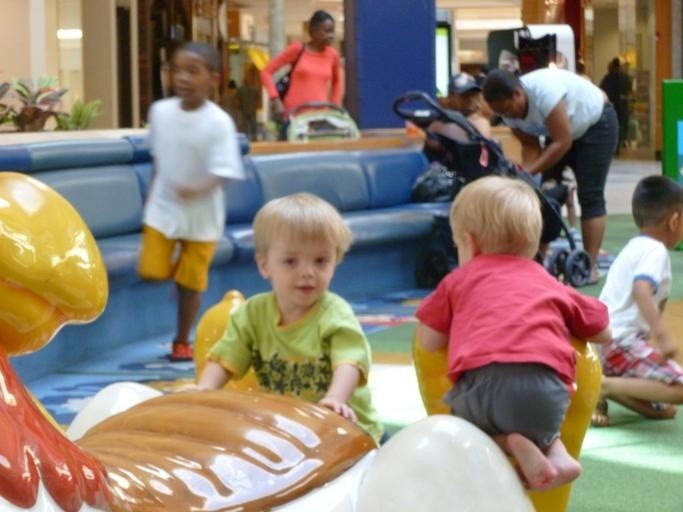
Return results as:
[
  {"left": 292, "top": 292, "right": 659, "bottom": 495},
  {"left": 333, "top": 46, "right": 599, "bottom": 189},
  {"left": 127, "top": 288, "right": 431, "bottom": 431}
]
[{"left": 0, "top": 134, "right": 449, "bottom": 384}]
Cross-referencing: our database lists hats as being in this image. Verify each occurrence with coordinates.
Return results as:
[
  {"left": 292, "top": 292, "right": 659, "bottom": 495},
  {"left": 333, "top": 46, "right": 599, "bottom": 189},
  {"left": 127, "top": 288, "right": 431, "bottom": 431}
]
[{"left": 448, "top": 72, "right": 481, "bottom": 94}]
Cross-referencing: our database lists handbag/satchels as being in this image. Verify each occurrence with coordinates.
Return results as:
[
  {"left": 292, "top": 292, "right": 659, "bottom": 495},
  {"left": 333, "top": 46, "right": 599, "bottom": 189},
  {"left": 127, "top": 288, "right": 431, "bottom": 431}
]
[
  {"left": 275, "top": 42, "right": 305, "bottom": 104},
  {"left": 410, "top": 168, "right": 457, "bottom": 202}
]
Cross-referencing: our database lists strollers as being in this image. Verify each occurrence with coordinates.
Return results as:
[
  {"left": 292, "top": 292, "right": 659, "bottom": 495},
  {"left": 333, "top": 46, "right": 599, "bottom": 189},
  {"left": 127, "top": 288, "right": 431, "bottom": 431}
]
[
  {"left": 393, "top": 90, "right": 590, "bottom": 288},
  {"left": 280, "top": 100, "right": 360, "bottom": 142}
]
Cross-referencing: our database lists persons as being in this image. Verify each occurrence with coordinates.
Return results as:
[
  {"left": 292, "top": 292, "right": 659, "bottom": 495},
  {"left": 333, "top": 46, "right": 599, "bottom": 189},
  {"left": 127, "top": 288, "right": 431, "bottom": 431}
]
[
  {"left": 478, "top": 67, "right": 622, "bottom": 287},
  {"left": 598, "top": 57, "right": 633, "bottom": 147},
  {"left": 136, "top": 40, "right": 247, "bottom": 360},
  {"left": 260, "top": 10, "right": 346, "bottom": 133},
  {"left": 168, "top": 189, "right": 386, "bottom": 447},
  {"left": 423, "top": 71, "right": 492, "bottom": 170},
  {"left": 559, "top": 167, "right": 580, "bottom": 237},
  {"left": 416, "top": 173, "right": 610, "bottom": 493},
  {"left": 589, "top": 171, "right": 683, "bottom": 425}
]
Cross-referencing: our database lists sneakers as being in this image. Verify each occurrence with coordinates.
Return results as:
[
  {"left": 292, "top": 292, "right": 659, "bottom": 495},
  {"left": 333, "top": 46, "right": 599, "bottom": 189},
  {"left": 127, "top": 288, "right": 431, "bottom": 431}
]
[{"left": 171, "top": 340, "right": 192, "bottom": 361}]
